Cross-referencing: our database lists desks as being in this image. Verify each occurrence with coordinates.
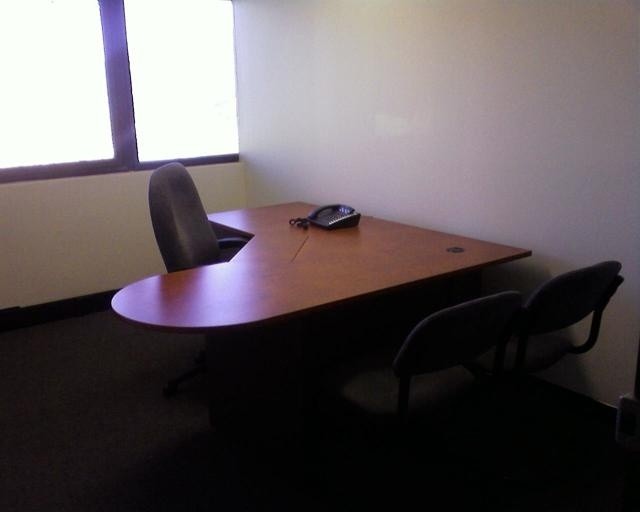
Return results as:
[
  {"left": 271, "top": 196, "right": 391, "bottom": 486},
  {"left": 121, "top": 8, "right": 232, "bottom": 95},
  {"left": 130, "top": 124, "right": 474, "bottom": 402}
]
[{"left": 111, "top": 200, "right": 534, "bottom": 511}]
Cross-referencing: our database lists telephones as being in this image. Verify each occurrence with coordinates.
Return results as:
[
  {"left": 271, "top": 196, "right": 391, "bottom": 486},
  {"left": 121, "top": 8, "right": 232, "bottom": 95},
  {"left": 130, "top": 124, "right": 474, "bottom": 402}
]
[{"left": 307, "top": 204, "right": 361, "bottom": 230}]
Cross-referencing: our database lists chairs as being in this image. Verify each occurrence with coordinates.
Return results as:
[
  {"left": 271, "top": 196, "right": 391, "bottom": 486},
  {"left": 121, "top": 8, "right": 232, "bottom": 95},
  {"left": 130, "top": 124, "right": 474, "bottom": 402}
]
[
  {"left": 340, "top": 288, "right": 525, "bottom": 510},
  {"left": 146, "top": 161, "right": 264, "bottom": 397},
  {"left": 473, "top": 259, "right": 624, "bottom": 459}
]
